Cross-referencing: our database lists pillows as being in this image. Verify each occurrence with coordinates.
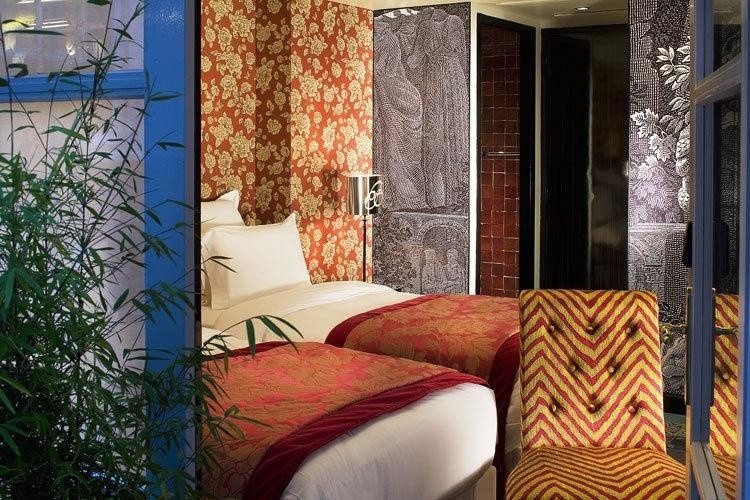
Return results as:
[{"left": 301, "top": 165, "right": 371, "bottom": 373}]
[{"left": 201, "top": 190, "right": 311, "bottom": 310}]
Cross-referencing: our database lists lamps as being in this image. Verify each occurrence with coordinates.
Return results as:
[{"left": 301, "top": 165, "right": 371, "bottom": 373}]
[{"left": 345, "top": 173, "right": 383, "bottom": 282}]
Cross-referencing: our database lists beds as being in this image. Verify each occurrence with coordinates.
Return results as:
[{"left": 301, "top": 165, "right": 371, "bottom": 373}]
[
  {"left": 201, "top": 325, "right": 498, "bottom": 499},
  {"left": 201, "top": 281, "right": 518, "bottom": 460}
]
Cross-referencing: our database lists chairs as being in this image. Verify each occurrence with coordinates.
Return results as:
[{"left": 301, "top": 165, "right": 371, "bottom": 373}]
[{"left": 507, "top": 285, "right": 690, "bottom": 500}]
